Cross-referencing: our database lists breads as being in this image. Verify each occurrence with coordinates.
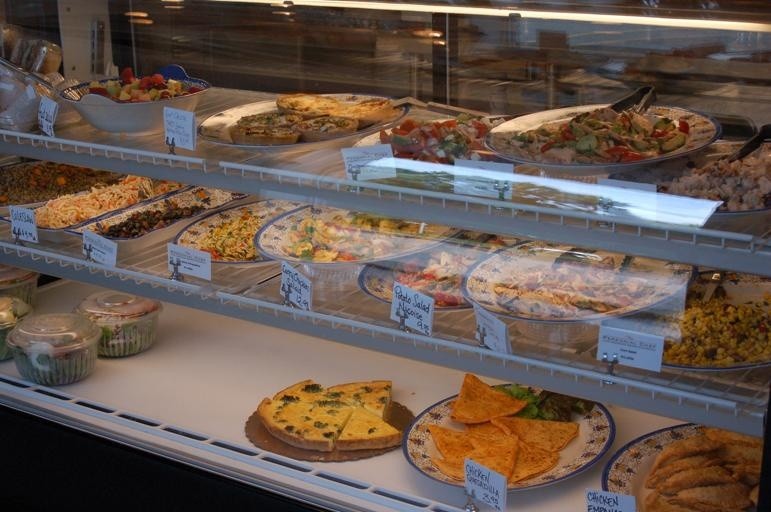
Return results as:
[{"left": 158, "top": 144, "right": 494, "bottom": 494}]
[{"left": 257, "top": 378, "right": 403, "bottom": 452}]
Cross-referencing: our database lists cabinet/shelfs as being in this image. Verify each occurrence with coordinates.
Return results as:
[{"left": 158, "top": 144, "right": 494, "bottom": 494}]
[{"left": 0, "top": 55, "right": 771, "bottom": 509}]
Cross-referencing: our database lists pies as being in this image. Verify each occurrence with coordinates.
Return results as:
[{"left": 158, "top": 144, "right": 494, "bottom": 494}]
[
  {"left": 229, "top": 93, "right": 394, "bottom": 143},
  {"left": 427, "top": 373, "right": 579, "bottom": 482}
]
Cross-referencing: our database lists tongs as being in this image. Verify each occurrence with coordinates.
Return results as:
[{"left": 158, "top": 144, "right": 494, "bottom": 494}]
[{"left": 570, "top": 84, "right": 659, "bottom": 134}]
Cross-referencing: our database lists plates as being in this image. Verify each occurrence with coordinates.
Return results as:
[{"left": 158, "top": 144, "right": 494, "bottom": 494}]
[
  {"left": 598, "top": 424, "right": 768, "bottom": 511},
  {"left": 358, "top": 239, "right": 538, "bottom": 312},
  {"left": 196, "top": 95, "right": 414, "bottom": 155},
  {"left": 1, "top": 151, "right": 253, "bottom": 255},
  {"left": 172, "top": 198, "right": 311, "bottom": 268},
  {"left": 622, "top": 268, "right": 771, "bottom": 368},
  {"left": 400, "top": 382, "right": 615, "bottom": 494},
  {"left": 242, "top": 398, "right": 416, "bottom": 463},
  {"left": 254, "top": 205, "right": 465, "bottom": 269},
  {"left": 343, "top": 101, "right": 771, "bottom": 218},
  {"left": 460, "top": 240, "right": 697, "bottom": 325}
]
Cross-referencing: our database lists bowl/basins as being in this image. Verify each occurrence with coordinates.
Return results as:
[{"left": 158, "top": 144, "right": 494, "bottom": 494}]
[
  {"left": 0, "top": 262, "right": 42, "bottom": 315},
  {"left": 1, "top": 20, "right": 64, "bottom": 92},
  {"left": 74, "top": 289, "right": 164, "bottom": 359},
  {"left": 5, "top": 310, "right": 102, "bottom": 389},
  {"left": 61, "top": 64, "right": 211, "bottom": 139},
  {"left": 0, "top": 294, "right": 34, "bottom": 365}
]
